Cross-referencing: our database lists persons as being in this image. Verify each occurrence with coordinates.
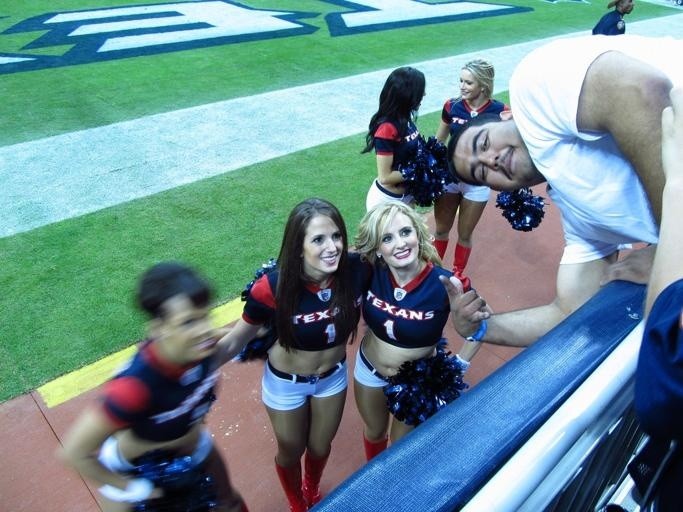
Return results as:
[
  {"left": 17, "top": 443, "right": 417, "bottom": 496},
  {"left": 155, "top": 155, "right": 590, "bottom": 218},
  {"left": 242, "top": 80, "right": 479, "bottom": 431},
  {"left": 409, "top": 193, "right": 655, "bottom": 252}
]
[
  {"left": 350, "top": 199, "right": 483, "bottom": 464},
  {"left": 429, "top": 58, "right": 510, "bottom": 293},
  {"left": 435, "top": 31, "right": 683, "bottom": 348},
  {"left": 360, "top": 65, "right": 425, "bottom": 213},
  {"left": 55, "top": 259, "right": 260, "bottom": 512},
  {"left": 210, "top": 197, "right": 374, "bottom": 512},
  {"left": 590, "top": 0, "right": 633, "bottom": 34},
  {"left": 630, "top": 87, "right": 683, "bottom": 438}
]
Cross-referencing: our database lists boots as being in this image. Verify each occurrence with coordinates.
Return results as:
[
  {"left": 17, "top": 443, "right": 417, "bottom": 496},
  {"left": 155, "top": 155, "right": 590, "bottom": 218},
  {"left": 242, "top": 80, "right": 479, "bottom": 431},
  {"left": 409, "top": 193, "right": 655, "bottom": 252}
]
[
  {"left": 452, "top": 244, "right": 472, "bottom": 292},
  {"left": 304, "top": 446, "right": 332, "bottom": 506},
  {"left": 273, "top": 455, "right": 309, "bottom": 511}
]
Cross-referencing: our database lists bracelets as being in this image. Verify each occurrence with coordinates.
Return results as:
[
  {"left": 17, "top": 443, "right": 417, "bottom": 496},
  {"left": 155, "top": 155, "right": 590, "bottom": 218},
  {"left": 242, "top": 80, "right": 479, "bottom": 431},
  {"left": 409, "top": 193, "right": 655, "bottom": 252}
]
[{"left": 461, "top": 317, "right": 488, "bottom": 344}]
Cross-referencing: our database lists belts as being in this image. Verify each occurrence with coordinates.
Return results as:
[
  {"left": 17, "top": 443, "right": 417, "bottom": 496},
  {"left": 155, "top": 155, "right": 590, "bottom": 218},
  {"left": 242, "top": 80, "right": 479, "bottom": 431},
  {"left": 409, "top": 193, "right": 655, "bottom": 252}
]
[
  {"left": 359, "top": 336, "right": 391, "bottom": 384},
  {"left": 376, "top": 179, "right": 410, "bottom": 199},
  {"left": 267, "top": 352, "right": 347, "bottom": 383}
]
